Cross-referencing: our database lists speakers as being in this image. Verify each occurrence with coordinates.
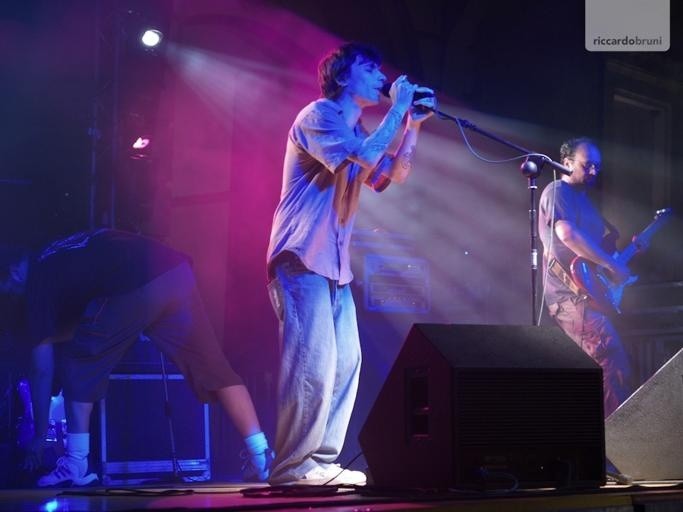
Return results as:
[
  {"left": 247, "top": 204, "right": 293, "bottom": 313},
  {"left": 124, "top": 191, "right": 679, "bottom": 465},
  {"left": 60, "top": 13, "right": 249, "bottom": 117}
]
[
  {"left": 360, "top": 320, "right": 607, "bottom": 490},
  {"left": 605, "top": 348, "right": 683, "bottom": 481}
]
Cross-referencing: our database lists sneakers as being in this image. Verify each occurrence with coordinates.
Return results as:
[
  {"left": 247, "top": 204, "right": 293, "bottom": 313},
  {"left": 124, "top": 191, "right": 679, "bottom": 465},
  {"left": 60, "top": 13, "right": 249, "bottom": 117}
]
[
  {"left": 238, "top": 448, "right": 367, "bottom": 487},
  {"left": 36, "top": 452, "right": 98, "bottom": 487}
]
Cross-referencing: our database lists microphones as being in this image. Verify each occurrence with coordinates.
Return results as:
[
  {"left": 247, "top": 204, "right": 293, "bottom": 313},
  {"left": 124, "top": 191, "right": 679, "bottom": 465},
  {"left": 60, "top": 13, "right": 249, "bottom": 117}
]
[{"left": 382, "top": 83, "right": 434, "bottom": 106}]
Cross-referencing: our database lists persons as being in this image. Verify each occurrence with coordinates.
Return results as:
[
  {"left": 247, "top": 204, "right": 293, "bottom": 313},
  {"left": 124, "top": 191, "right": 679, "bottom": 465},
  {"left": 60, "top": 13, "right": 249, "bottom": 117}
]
[
  {"left": 538, "top": 138, "right": 650, "bottom": 484},
  {"left": 0, "top": 228, "right": 277, "bottom": 487},
  {"left": 266, "top": 42, "right": 437, "bottom": 487}
]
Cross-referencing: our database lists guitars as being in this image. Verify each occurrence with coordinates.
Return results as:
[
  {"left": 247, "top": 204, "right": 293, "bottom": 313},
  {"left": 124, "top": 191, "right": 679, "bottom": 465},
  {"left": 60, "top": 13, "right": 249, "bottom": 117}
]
[
  {"left": 570, "top": 206, "right": 671, "bottom": 314},
  {"left": 12, "top": 380, "right": 111, "bottom": 486}
]
